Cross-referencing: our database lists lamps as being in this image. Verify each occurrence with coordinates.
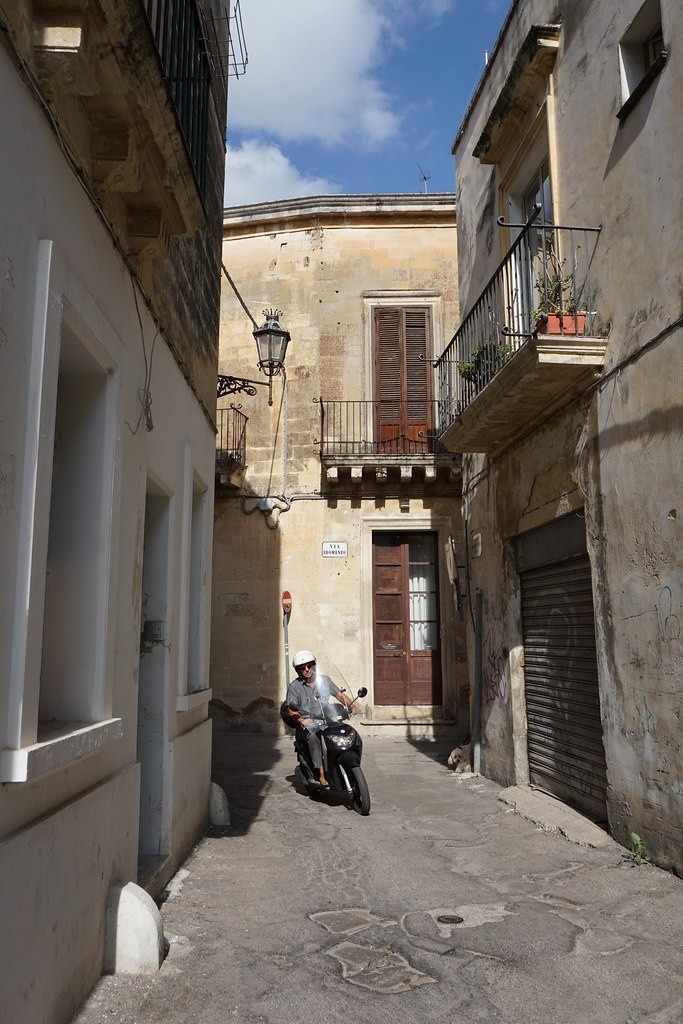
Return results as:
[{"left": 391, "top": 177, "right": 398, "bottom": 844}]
[{"left": 216, "top": 308, "right": 291, "bottom": 406}]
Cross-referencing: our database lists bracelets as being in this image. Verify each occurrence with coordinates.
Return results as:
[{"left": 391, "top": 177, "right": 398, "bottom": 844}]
[{"left": 351, "top": 706, "right": 355, "bottom": 708}]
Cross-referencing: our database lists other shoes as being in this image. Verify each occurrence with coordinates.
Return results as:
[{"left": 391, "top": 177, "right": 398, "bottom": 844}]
[{"left": 318, "top": 773, "right": 330, "bottom": 786}]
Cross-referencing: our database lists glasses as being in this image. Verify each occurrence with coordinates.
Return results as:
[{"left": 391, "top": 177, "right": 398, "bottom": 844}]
[{"left": 300, "top": 664, "right": 314, "bottom": 671}]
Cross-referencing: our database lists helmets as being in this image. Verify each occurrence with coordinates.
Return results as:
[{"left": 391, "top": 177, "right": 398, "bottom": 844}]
[{"left": 292, "top": 649, "right": 316, "bottom": 667}]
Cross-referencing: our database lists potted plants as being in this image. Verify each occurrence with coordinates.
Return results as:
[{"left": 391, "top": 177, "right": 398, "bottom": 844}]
[
  {"left": 456, "top": 337, "right": 512, "bottom": 392},
  {"left": 535, "top": 244, "right": 586, "bottom": 335}
]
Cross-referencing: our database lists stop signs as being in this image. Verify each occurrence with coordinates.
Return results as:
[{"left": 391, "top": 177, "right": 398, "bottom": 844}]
[{"left": 281, "top": 591, "right": 292, "bottom": 613}]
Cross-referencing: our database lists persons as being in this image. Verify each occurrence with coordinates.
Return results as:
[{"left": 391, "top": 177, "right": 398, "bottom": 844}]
[{"left": 286, "top": 650, "right": 360, "bottom": 786}]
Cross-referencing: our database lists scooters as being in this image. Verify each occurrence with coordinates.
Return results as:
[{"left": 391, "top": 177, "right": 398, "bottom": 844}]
[{"left": 282, "top": 688, "right": 371, "bottom": 815}]
